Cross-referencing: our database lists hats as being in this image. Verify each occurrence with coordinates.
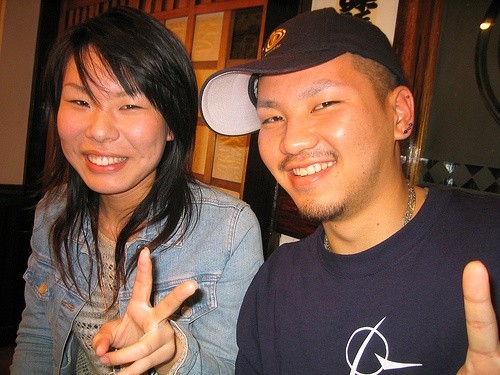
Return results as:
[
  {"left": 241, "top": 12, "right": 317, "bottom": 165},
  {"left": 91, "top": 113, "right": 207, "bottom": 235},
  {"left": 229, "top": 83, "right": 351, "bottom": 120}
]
[{"left": 200, "top": 8, "right": 402, "bottom": 138}]
